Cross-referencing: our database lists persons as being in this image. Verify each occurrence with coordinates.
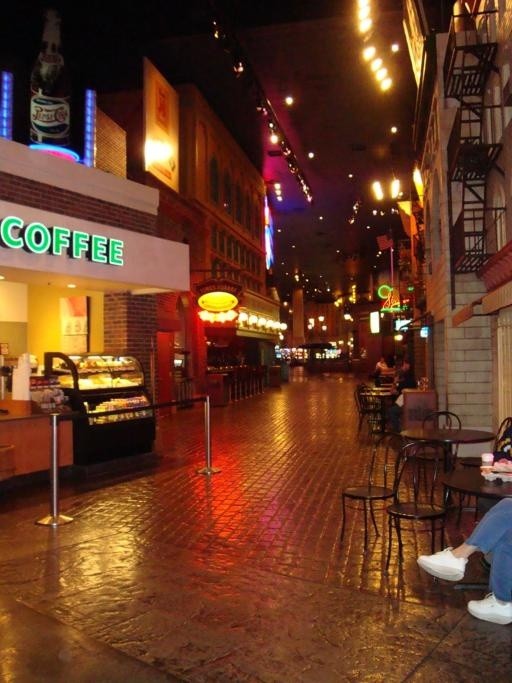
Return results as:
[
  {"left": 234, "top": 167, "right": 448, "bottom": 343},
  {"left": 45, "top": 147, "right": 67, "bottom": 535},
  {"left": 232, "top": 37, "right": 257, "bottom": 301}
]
[
  {"left": 394, "top": 360, "right": 416, "bottom": 392},
  {"left": 375, "top": 356, "right": 388, "bottom": 371},
  {"left": 416, "top": 496, "right": 511, "bottom": 626}
]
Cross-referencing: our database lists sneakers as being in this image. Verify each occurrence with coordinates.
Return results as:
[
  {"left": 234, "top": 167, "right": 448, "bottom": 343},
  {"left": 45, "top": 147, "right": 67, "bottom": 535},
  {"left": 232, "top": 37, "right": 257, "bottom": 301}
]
[
  {"left": 416, "top": 545, "right": 468, "bottom": 580},
  {"left": 467, "top": 591, "right": 512, "bottom": 624}
]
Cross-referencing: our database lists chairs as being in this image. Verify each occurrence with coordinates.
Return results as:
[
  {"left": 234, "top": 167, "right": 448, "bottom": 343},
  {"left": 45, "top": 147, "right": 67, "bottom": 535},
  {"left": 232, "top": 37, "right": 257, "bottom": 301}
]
[{"left": 338, "top": 381, "right": 512, "bottom": 580}]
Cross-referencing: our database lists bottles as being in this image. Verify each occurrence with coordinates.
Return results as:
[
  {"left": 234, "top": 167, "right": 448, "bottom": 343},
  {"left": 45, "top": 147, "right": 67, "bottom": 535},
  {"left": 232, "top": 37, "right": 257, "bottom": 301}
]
[{"left": 28, "top": 7, "right": 72, "bottom": 149}]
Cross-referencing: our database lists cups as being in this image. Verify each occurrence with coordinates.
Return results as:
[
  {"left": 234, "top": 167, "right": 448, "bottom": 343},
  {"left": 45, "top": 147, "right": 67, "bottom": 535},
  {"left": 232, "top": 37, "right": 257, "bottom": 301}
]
[{"left": 480, "top": 452, "right": 494, "bottom": 465}]
